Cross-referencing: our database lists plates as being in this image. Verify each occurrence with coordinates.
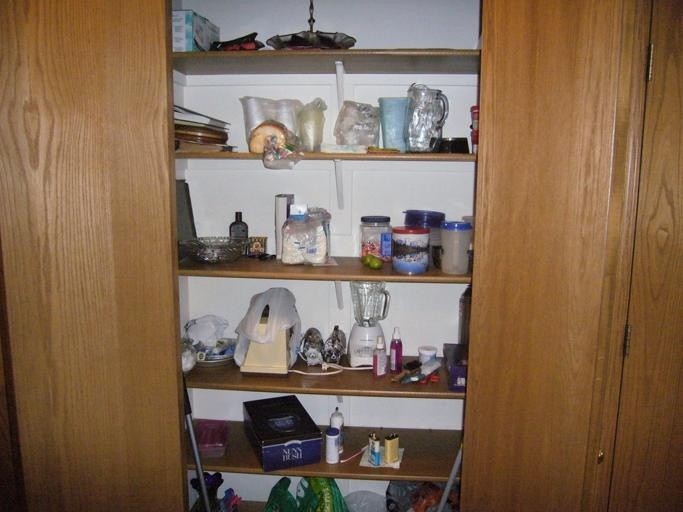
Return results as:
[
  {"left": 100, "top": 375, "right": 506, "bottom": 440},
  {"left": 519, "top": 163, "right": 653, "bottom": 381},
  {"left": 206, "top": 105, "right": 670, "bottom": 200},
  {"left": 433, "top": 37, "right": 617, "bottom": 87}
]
[
  {"left": 198, "top": 358, "right": 234, "bottom": 364},
  {"left": 266, "top": 31, "right": 356, "bottom": 50}
]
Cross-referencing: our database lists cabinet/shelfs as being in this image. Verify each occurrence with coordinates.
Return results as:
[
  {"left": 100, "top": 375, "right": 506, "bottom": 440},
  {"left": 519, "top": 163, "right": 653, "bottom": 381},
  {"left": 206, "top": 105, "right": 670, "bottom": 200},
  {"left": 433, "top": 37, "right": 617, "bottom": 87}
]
[{"left": 164, "top": 0, "right": 482, "bottom": 512}]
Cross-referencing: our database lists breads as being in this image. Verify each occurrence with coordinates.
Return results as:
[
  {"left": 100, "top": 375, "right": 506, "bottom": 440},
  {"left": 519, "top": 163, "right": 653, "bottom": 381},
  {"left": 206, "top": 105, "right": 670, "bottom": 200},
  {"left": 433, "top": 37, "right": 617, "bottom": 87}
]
[{"left": 248, "top": 123, "right": 287, "bottom": 153}]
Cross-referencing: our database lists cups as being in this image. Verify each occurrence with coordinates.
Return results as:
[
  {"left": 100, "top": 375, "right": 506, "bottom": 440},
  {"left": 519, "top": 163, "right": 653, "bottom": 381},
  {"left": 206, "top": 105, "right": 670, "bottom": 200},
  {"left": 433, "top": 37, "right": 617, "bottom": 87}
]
[
  {"left": 378, "top": 83, "right": 449, "bottom": 153},
  {"left": 440, "top": 216, "right": 473, "bottom": 274}
]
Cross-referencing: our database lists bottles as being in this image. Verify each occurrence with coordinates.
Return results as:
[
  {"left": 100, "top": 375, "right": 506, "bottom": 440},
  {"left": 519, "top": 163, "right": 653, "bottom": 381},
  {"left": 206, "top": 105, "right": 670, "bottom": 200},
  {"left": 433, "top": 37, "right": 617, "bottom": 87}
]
[
  {"left": 418, "top": 346, "right": 437, "bottom": 364},
  {"left": 281, "top": 205, "right": 331, "bottom": 265},
  {"left": 367, "top": 432, "right": 400, "bottom": 466},
  {"left": 372, "top": 327, "right": 402, "bottom": 379},
  {"left": 230, "top": 212, "right": 248, "bottom": 256},
  {"left": 325, "top": 406, "right": 344, "bottom": 464}
]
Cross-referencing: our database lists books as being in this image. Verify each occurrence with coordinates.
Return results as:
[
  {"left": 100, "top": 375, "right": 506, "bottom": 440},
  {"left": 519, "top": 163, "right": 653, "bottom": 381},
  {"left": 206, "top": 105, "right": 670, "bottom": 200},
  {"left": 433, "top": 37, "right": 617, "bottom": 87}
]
[{"left": 173, "top": 103, "right": 238, "bottom": 154}]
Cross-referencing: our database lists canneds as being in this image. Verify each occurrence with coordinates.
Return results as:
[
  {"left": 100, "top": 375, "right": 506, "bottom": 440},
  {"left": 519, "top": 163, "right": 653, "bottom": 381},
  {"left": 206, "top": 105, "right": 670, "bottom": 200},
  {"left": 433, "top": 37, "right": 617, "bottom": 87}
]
[{"left": 391, "top": 225, "right": 430, "bottom": 276}]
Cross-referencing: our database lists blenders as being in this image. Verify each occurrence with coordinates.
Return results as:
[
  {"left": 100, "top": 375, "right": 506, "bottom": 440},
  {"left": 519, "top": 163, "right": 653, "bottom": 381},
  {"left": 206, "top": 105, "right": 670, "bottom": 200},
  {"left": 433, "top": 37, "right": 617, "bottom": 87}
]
[{"left": 347, "top": 280, "right": 391, "bottom": 369}]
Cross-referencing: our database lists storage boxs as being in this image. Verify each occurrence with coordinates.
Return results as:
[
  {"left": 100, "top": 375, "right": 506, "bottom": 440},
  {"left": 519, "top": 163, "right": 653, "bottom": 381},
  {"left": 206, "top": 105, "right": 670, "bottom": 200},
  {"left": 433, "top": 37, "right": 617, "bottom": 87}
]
[{"left": 240, "top": 394, "right": 324, "bottom": 473}]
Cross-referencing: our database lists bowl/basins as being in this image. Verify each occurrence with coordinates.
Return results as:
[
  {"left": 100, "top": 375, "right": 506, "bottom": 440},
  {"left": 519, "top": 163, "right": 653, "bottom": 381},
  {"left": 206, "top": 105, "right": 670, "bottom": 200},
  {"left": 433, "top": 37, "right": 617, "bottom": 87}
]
[{"left": 179, "top": 237, "right": 251, "bottom": 263}]
